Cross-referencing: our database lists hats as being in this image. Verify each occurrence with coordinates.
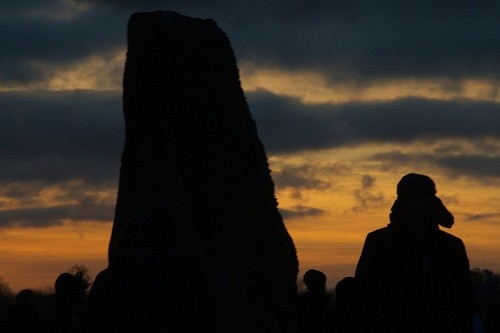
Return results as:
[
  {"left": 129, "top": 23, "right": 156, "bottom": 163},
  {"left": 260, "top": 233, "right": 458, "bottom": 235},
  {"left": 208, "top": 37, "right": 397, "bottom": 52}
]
[{"left": 390, "top": 173, "right": 456, "bottom": 230}]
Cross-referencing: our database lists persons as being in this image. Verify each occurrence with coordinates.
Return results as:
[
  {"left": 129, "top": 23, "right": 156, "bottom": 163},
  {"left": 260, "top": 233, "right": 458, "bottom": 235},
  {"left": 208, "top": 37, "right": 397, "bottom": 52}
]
[{"left": 14, "top": 172, "right": 500, "bottom": 333}]
